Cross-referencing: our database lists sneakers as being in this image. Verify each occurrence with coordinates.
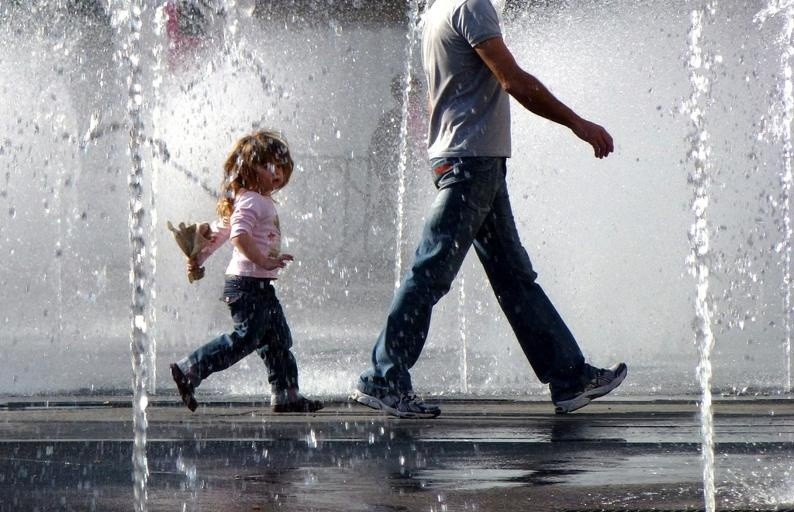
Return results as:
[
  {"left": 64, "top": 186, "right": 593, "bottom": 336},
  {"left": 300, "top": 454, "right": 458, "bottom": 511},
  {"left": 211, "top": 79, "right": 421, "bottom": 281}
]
[
  {"left": 170, "top": 363, "right": 200, "bottom": 412},
  {"left": 549, "top": 362, "right": 627, "bottom": 414},
  {"left": 349, "top": 376, "right": 441, "bottom": 419},
  {"left": 272, "top": 397, "right": 324, "bottom": 412}
]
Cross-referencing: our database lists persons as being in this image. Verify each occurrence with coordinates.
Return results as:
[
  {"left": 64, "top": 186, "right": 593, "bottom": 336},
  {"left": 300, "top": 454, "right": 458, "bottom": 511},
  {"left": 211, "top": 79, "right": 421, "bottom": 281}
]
[
  {"left": 365, "top": 72, "right": 433, "bottom": 285},
  {"left": 168, "top": 130, "right": 327, "bottom": 412},
  {"left": 350, "top": 0, "right": 628, "bottom": 418}
]
